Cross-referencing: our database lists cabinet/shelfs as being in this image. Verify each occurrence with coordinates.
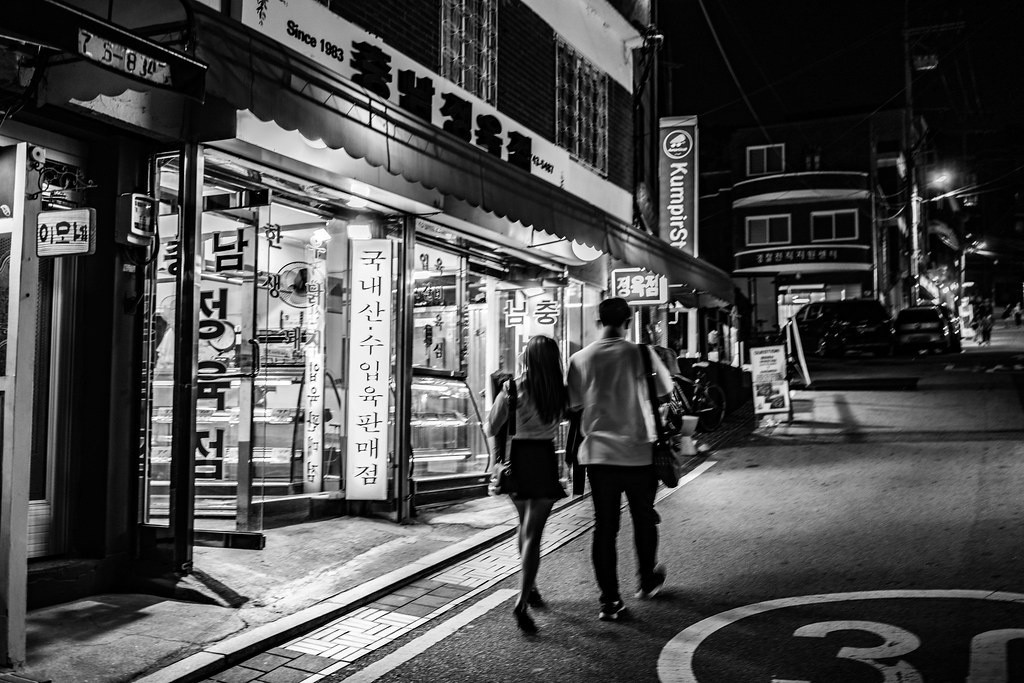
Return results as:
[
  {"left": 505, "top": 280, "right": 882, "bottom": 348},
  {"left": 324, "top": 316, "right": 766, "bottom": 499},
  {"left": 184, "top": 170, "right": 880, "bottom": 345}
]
[
  {"left": 149, "top": 366, "right": 341, "bottom": 496},
  {"left": 410, "top": 364, "right": 494, "bottom": 495}
]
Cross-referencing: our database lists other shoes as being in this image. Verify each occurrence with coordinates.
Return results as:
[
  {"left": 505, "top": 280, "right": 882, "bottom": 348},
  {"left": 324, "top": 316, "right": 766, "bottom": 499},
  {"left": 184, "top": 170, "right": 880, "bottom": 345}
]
[
  {"left": 599, "top": 599, "right": 625, "bottom": 620},
  {"left": 638, "top": 567, "right": 666, "bottom": 598},
  {"left": 529, "top": 589, "right": 543, "bottom": 607},
  {"left": 512, "top": 603, "right": 536, "bottom": 636}
]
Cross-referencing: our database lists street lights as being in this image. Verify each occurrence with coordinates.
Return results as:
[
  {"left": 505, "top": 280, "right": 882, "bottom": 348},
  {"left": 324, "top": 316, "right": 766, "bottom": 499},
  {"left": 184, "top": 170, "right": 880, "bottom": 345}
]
[{"left": 908, "top": 173, "right": 949, "bottom": 303}]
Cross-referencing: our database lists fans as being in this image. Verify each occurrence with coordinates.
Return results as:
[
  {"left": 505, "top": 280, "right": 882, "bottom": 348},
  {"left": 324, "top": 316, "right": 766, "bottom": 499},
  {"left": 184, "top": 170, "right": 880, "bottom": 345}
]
[{"left": 276, "top": 261, "right": 325, "bottom": 309}]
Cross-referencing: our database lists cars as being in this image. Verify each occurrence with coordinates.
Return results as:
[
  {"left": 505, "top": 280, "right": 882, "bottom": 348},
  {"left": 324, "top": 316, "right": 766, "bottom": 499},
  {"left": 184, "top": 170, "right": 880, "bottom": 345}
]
[
  {"left": 895, "top": 306, "right": 951, "bottom": 358},
  {"left": 936, "top": 304, "right": 962, "bottom": 353}
]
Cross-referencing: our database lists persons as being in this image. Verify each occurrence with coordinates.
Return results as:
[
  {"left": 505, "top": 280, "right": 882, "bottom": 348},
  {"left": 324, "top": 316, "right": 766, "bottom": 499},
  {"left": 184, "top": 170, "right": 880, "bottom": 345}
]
[
  {"left": 566, "top": 298, "right": 674, "bottom": 622},
  {"left": 1001, "top": 303, "right": 1012, "bottom": 329},
  {"left": 483, "top": 336, "right": 568, "bottom": 632},
  {"left": 1013, "top": 302, "right": 1022, "bottom": 329},
  {"left": 973, "top": 297, "right": 995, "bottom": 346},
  {"left": 708, "top": 325, "right": 719, "bottom": 348}
]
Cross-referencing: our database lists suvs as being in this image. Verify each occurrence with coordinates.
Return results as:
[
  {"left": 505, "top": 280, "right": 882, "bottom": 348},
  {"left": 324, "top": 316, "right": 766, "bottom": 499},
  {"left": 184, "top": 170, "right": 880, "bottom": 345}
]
[{"left": 780, "top": 298, "right": 899, "bottom": 369}]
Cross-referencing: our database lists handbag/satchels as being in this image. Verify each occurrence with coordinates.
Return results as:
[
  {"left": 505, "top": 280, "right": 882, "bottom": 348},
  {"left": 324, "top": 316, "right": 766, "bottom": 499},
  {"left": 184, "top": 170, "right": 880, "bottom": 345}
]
[
  {"left": 653, "top": 438, "right": 679, "bottom": 488},
  {"left": 488, "top": 463, "right": 513, "bottom": 496}
]
